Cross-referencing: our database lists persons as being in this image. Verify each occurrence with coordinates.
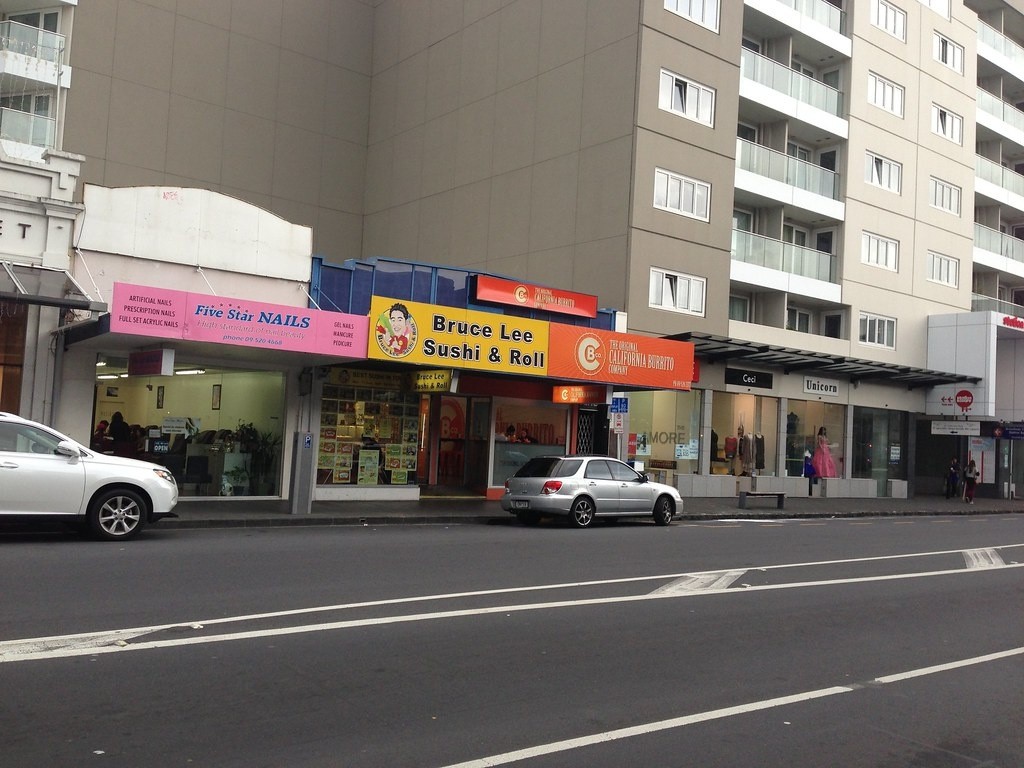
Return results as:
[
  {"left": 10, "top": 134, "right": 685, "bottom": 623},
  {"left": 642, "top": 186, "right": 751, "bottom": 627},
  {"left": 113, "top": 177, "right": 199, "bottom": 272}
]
[
  {"left": 499, "top": 424, "right": 534, "bottom": 444},
  {"left": 93, "top": 411, "right": 132, "bottom": 458},
  {"left": 811, "top": 426, "right": 838, "bottom": 477},
  {"left": 803, "top": 449, "right": 817, "bottom": 477},
  {"left": 711, "top": 427, "right": 764, "bottom": 475},
  {"left": 961, "top": 460, "right": 980, "bottom": 504},
  {"left": 946, "top": 456, "right": 962, "bottom": 500}
]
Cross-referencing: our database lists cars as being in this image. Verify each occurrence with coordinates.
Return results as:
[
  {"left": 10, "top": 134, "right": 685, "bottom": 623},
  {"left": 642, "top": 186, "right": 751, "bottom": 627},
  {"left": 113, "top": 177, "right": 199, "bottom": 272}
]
[{"left": 499, "top": 455, "right": 684, "bottom": 528}]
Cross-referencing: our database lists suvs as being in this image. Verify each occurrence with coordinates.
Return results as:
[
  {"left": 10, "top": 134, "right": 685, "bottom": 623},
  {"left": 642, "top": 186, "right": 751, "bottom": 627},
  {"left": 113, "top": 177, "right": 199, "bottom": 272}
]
[{"left": 0, "top": 411, "right": 179, "bottom": 542}]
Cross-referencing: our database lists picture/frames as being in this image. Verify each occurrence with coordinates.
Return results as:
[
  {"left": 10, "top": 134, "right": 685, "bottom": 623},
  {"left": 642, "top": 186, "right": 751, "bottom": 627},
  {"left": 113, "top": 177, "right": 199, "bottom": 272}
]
[{"left": 212, "top": 384, "right": 222, "bottom": 410}]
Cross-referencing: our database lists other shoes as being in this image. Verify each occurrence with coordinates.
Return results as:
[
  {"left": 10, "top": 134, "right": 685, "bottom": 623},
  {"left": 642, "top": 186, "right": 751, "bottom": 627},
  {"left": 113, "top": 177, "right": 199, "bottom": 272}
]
[
  {"left": 970, "top": 502, "right": 973, "bottom": 505},
  {"left": 966, "top": 497, "right": 969, "bottom": 502}
]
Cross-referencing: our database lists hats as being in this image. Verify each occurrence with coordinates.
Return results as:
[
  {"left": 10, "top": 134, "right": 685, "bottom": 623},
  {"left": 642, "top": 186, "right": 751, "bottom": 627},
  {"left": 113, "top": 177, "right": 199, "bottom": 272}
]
[{"left": 97, "top": 424, "right": 106, "bottom": 430}]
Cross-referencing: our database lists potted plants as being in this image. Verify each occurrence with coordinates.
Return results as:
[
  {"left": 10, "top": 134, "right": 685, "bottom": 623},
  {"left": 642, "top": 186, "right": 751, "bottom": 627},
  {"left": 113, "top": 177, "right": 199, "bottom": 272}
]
[{"left": 175, "top": 422, "right": 283, "bottom": 495}]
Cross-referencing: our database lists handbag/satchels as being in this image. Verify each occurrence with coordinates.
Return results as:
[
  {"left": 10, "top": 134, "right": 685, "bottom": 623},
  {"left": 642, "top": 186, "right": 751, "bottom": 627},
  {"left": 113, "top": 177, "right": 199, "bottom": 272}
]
[{"left": 726, "top": 452, "right": 735, "bottom": 459}]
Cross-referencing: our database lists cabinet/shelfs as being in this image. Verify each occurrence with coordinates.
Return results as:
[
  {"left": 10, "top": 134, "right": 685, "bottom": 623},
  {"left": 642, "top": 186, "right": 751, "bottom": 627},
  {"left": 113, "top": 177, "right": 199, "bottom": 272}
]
[{"left": 184, "top": 443, "right": 252, "bottom": 494}]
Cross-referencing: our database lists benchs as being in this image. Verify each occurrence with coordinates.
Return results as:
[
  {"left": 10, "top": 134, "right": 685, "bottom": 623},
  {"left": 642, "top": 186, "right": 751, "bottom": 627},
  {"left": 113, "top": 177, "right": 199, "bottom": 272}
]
[{"left": 739, "top": 491, "right": 786, "bottom": 510}]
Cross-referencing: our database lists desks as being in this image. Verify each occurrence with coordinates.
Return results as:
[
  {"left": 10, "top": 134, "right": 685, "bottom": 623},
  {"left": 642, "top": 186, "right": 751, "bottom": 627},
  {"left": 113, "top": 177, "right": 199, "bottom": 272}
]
[{"left": 440, "top": 438, "right": 539, "bottom": 489}]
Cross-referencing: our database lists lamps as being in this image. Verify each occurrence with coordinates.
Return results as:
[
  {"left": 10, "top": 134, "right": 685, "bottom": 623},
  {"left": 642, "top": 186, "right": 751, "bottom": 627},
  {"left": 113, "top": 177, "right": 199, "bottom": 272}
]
[
  {"left": 97, "top": 375, "right": 119, "bottom": 380},
  {"left": 175, "top": 369, "right": 205, "bottom": 375}
]
